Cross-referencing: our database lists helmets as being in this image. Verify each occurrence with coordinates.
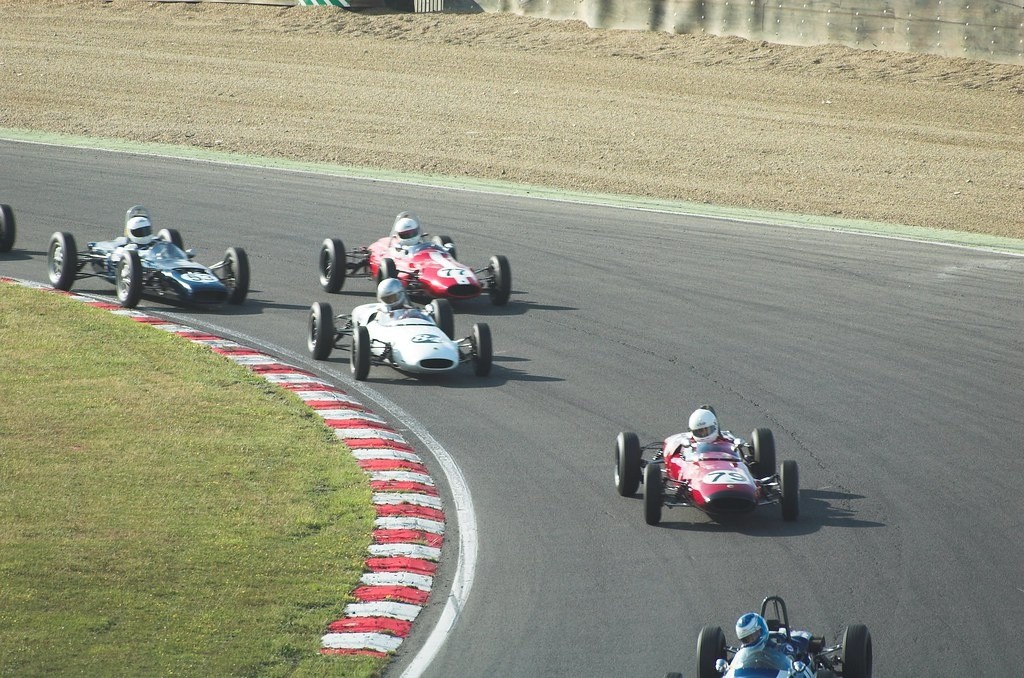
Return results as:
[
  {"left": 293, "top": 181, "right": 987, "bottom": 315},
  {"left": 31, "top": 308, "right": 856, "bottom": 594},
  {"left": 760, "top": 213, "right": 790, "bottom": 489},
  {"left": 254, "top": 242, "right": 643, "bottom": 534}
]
[
  {"left": 395, "top": 218, "right": 420, "bottom": 246},
  {"left": 689, "top": 409, "right": 719, "bottom": 443},
  {"left": 735, "top": 613, "right": 770, "bottom": 652},
  {"left": 127, "top": 216, "right": 152, "bottom": 244},
  {"left": 376, "top": 277, "right": 406, "bottom": 312}
]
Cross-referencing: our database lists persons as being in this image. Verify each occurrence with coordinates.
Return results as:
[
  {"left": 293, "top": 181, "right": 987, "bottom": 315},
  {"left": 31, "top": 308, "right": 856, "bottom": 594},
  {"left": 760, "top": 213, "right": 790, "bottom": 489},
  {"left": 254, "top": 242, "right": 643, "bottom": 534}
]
[
  {"left": 376, "top": 277, "right": 432, "bottom": 321},
  {"left": 679, "top": 409, "right": 745, "bottom": 455},
  {"left": 394, "top": 218, "right": 423, "bottom": 254},
  {"left": 715, "top": 612, "right": 798, "bottom": 661},
  {"left": 115, "top": 217, "right": 164, "bottom": 250}
]
